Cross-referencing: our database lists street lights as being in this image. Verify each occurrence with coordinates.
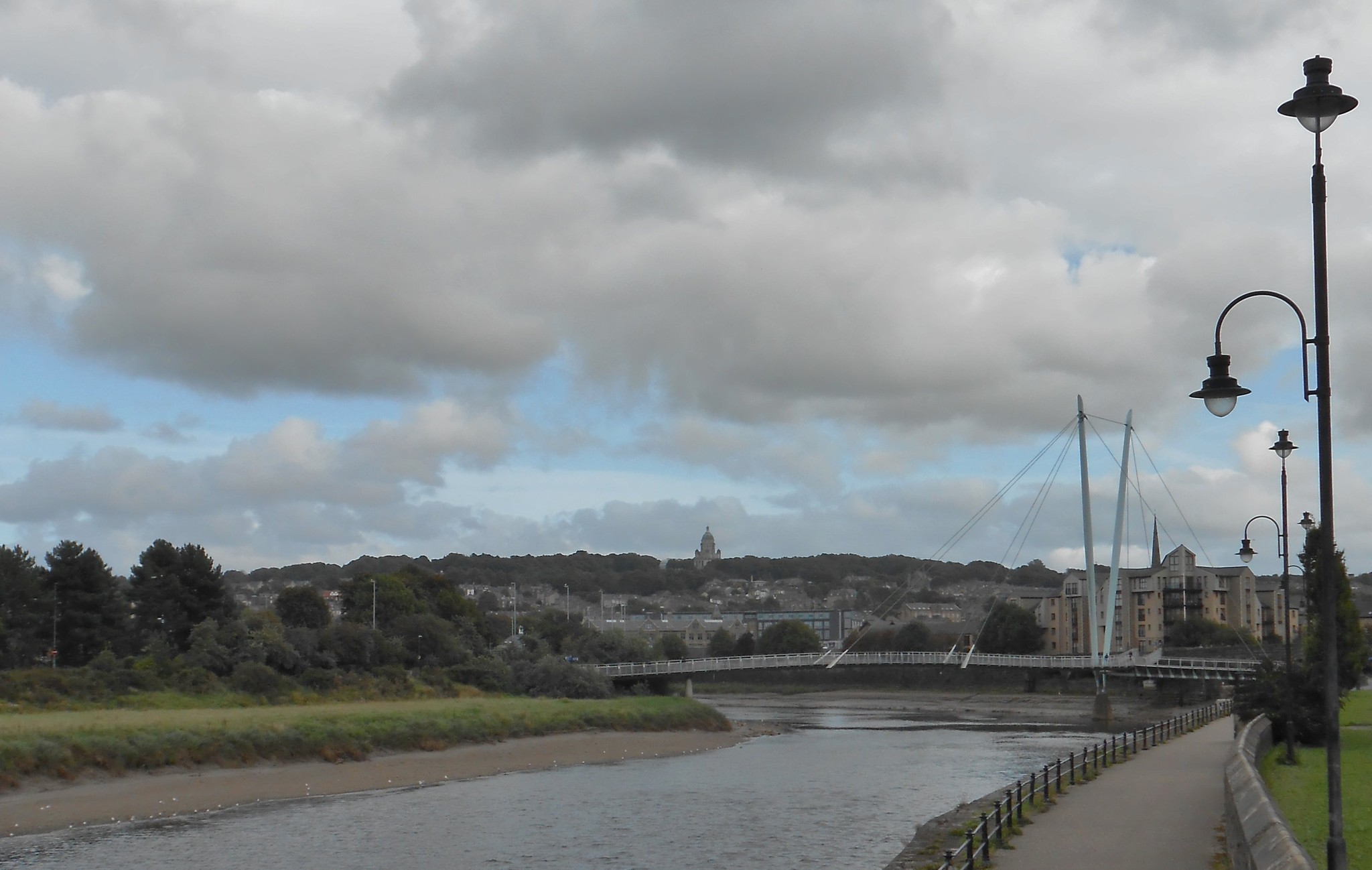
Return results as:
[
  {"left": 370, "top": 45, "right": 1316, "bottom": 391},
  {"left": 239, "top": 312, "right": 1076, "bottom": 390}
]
[
  {"left": 564, "top": 585, "right": 569, "bottom": 624},
  {"left": 598, "top": 590, "right": 604, "bottom": 633},
  {"left": 1233, "top": 427, "right": 1300, "bottom": 764},
  {"left": 1279, "top": 509, "right": 1316, "bottom": 709},
  {"left": 511, "top": 582, "right": 516, "bottom": 635},
  {"left": 1184, "top": 55, "right": 1361, "bottom": 870},
  {"left": 510, "top": 617, "right": 514, "bottom": 637},
  {"left": 370, "top": 580, "right": 376, "bottom": 629},
  {"left": 417, "top": 635, "right": 423, "bottom": 669}
]
